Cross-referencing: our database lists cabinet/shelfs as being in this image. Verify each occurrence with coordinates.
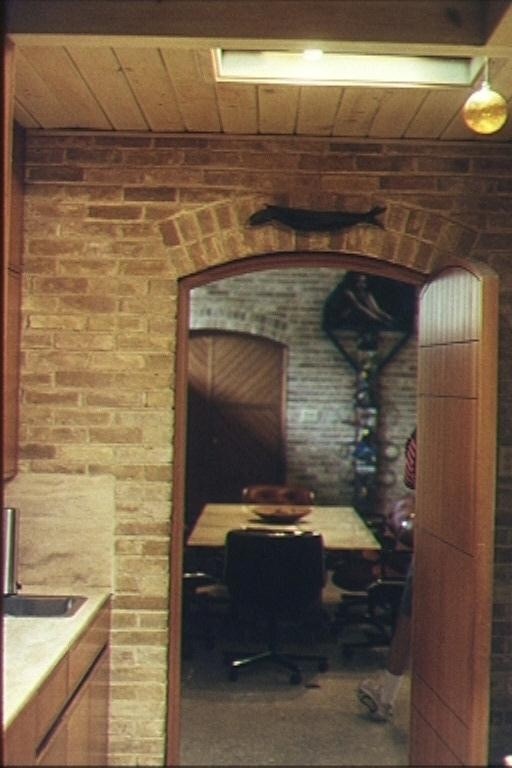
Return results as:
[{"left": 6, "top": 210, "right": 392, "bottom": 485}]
[{"left": 2, "top": 584, "right": 114, "bottom": 765}]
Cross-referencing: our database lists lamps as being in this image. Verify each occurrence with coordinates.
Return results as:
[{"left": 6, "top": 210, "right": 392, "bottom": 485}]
[{"left": 462, "top": 57, "right": 510, "bottom": 136}]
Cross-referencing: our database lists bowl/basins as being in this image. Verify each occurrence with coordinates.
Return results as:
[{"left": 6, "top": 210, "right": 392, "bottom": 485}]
[{"left": 242, "top": 484, "right": 316, "bottom": 524}]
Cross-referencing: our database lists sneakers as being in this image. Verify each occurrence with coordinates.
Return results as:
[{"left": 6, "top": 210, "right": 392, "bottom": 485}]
[{"left": 358, "top": 678, "right": 392, "bottom": 720}]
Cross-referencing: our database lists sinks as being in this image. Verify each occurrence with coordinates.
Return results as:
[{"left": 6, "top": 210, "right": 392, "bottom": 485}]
[{"left": 0, "top": 594, "right": 89, "bottom": 618}]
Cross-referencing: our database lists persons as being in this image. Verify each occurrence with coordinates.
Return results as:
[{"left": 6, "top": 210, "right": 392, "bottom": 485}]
[
  {"left": 354, "top": 427, "right": 417, "bottom": 720},
  {"left": 340, "top": 274, "right": 392, "bottom": 351}
]
